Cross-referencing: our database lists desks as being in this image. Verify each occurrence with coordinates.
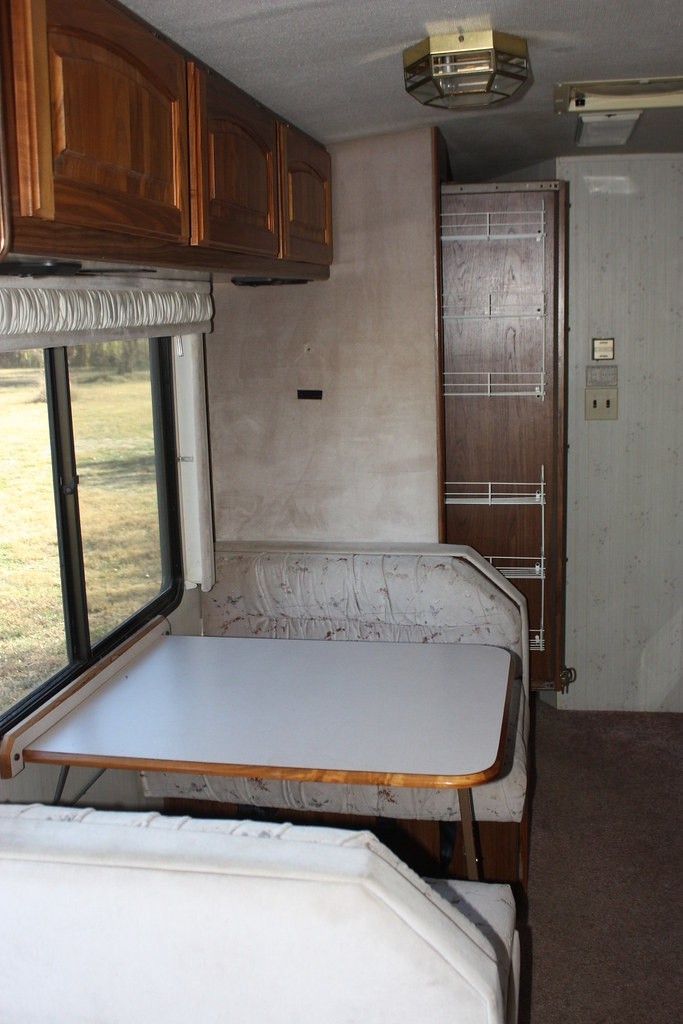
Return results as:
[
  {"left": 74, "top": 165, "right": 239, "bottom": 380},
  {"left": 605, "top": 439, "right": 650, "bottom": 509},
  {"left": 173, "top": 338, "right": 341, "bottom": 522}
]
[{"left": 22, "top": 633, "right": 518, "bottom": 879}]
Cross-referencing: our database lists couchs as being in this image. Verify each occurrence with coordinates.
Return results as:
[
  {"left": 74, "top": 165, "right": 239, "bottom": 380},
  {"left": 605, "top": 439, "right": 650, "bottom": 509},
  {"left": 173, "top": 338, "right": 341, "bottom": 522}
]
[
  {"left": 141, "top": 538, "right": 531, "bottom": 874},
  {"left": 0, "top": 803, "right": 521, "bottom": 1024}
]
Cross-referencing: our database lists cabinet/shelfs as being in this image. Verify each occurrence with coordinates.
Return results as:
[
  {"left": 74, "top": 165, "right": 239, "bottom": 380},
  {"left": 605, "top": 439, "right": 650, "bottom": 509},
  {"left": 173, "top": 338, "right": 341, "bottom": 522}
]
[
  {"left": 1, "top": 0, "right": 335, "bottom": 287},
  {"left": 439, "top": 180, "right": 569, "bottom": 693}
]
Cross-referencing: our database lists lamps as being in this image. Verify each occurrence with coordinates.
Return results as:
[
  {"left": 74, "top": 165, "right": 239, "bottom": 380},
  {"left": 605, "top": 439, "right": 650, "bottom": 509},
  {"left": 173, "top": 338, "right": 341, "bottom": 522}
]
[{"left": 402, "top": 30, "right": 533, "bottom": 113}]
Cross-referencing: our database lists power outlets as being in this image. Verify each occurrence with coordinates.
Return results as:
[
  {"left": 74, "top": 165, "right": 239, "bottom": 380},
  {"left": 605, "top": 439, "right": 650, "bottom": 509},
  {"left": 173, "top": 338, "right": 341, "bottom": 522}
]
[{"left": 584, "top": 388, "right": 618, "bottom": 420}]
